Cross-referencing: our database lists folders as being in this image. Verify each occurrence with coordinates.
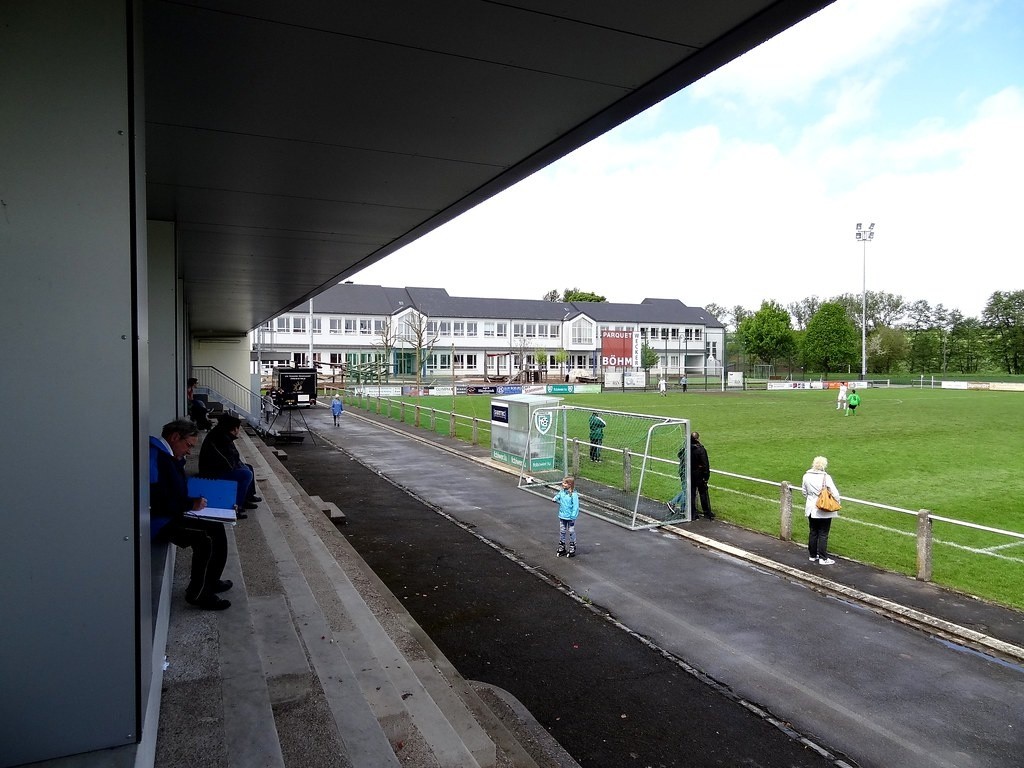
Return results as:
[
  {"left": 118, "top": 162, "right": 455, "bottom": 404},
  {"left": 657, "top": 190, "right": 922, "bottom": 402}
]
[{"left": 184, "top": 476, "right": 238, "bottom": 524}]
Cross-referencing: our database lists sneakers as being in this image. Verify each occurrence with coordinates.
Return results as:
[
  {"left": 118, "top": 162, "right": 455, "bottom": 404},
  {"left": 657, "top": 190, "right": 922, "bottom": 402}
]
[
  {"left": 819, "top": 558, "right": 835, "bottom": 565},
  {"left": 679, "top": 508, "right": 685, "bottom": 514},
  {"left": 667, "top": 501, "right": 675, "bottom": 514},
  {"left": 809, "top": 554, "right": 819, "bottom": 562}
]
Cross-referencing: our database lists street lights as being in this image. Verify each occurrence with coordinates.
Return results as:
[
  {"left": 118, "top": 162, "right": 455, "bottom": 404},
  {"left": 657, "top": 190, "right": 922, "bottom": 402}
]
[{"left": 856, "top": 222, "right": 875, "bottom": 380}]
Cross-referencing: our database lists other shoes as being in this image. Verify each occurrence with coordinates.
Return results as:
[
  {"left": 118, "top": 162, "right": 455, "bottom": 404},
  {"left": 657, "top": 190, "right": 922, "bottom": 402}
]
[
  {"left": 236, "top": 508, "right": 247, "bottom": 519},
  {"left": 692, "top": 514, "right": 700, "bottom": 520},
  {"left": 244, "top": 502, "right": 258, "bottom": 509},
  {"left": 337, "top": 424, "right": 339, "bottom": 427},
  {"left": 215, "top": 580, "right": 233, "bottom": 594},
  {"left": 186, "top": 592, "right": 231, "bottom": 610},
  {"left": 206, "top": 407, "right": 214, "bottom": 413},
  {"left": 334, "top": 423, "right": 336, "bottom": 426},
  {"left": 704, "top": 512, "right": 716, "bottom": 518},
  {"left": 590, "top": 458, "right": 603, "bottom": 462},
  {"left": 250, "top": 496, "right": 262, "bottom": 502}
]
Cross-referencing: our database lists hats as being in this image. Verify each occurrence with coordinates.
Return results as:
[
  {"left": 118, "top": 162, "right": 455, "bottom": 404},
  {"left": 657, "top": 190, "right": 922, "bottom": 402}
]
[{"left": 335, "top": 394, "right": 339, "bottom": 398}]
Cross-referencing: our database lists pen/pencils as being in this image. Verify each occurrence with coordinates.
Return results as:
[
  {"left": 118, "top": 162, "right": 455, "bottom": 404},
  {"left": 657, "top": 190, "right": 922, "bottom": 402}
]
[{"left": 198, "top": 494, "right": 203, "bottom": 498}]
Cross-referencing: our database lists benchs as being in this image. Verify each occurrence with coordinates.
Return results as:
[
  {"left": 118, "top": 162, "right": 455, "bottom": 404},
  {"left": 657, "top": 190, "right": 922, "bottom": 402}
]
[{"left": 487, "top": 376, "right": 504, "bottom": 381}]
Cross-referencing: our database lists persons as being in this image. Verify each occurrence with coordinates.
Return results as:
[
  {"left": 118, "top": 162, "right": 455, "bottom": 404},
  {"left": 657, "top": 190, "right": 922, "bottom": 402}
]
[
  {"left": 262, "top": 387, "right": 286, "bottom": 424},
  {"left": 680, "top": 374, "right": 689, "bottom": 393},
  {"left": 845, "top": 389, "right": 861, "bottom": 417},
  {"left": 331, "top": 393, "right": 344, "bottom": 428},
  {"left": 199, "top": 413, "right": 262, "bottom": 520},
  {"left": 551, "top": 477, "right": 579, "bottom": 560},
  {"left": 836, "top": 381, "right": 847, "bottom": 411},
  {"left": 588, "top": 411, "right": 606, "bottom": 463},
  {"left": 801, "top": 457, "right": 841, "bottom": 565},
  {"left": 657, "top": 377, "right": 669, "bottom": 397},
  {"left": 149, "top": 419, "right": 238, "bottom": 612},
  {"left": 187, "top": 377, "right": 214, "bottom": 420},
  {"left": 666, "top": 432, "right": 717, "bottom": 520}
]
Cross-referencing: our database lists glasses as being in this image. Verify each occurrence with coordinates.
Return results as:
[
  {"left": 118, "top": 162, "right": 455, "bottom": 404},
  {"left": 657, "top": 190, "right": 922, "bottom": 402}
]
[
  {"left": 183, "top": 438, "right": 195, "bottom": 450},
  {"left": 196, "top": 382, "right": 199, "bottom": 384}
]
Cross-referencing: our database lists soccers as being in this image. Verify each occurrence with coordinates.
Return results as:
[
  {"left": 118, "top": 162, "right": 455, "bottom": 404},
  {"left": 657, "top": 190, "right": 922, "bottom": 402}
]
[{"left": 525, "top": 476, "right": 535, "bottom": 484}]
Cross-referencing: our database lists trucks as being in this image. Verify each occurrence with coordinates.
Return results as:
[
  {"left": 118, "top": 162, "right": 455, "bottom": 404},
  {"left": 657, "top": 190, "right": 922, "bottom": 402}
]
[{"left": 271, "top": 366, "right": 318, "bottom": 409}]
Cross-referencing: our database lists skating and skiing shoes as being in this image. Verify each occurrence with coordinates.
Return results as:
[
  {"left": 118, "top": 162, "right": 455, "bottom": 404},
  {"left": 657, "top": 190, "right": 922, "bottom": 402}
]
[
  {"left": 566, "top": 542, "right": 578, "bottom": 558},
  {"left": 556, "top": 540, "right": 567, "bottom": 558}
]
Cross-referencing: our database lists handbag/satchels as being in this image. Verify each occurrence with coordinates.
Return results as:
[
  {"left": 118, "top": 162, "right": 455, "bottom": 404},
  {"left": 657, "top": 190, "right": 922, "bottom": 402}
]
[{"left": 816, "top": 473, "right": 842, "bottom": 512}]
